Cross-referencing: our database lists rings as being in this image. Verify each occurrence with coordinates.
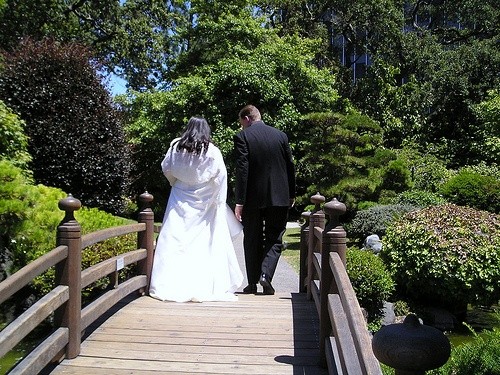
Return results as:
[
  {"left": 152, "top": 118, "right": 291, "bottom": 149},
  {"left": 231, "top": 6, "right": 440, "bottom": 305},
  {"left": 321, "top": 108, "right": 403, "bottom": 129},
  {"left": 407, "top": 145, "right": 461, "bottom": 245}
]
[{"left": 236, "top": 213, "right": 238, "bottom": 215}]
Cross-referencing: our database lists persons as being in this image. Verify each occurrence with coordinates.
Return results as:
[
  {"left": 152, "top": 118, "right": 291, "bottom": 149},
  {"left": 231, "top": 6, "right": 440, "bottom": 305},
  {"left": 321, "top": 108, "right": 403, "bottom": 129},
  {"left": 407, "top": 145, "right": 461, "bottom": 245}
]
[
  {"left": 148, "top": 116, "right": 250, "bottom": 303},
  {"left": 233, "top": 105, "right": 297, "bottom": 295}
]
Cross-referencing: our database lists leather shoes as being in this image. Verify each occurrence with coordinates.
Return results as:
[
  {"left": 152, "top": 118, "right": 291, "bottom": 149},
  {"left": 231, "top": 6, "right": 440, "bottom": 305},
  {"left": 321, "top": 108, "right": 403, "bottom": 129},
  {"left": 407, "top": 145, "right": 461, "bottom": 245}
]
[
  {"left": 243, "top": 284, "right": 257, "bottom": 293},
  {"left": 261, "top": 272, "right": 276, "bottom": 294}
]
